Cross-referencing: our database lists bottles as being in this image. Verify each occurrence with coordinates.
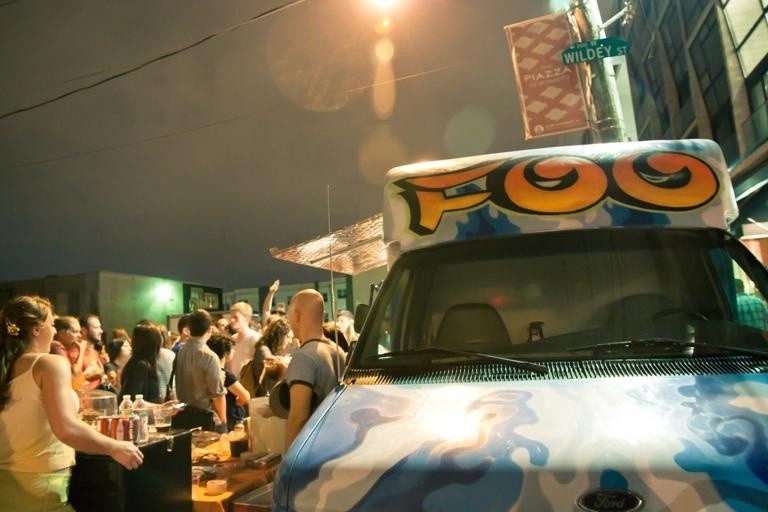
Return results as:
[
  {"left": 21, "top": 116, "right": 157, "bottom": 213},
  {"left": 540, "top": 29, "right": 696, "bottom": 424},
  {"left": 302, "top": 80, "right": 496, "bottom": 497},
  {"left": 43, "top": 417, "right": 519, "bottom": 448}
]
[
  {"left": 117, "top": 394, "right": 149, "bottom": 443},
  {"left": 229, "top": 424, "right": 249, "bottom": 460}
]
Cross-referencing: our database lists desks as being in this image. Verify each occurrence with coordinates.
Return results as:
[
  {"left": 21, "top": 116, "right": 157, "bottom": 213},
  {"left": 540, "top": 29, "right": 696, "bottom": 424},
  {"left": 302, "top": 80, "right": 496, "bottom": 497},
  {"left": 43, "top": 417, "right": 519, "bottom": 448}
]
[
  {"left": 69, "top": 425, "right": 202, "bottom": 511},
  {"left": 189, "top": 420, "right": 281, "bottom": 512}
]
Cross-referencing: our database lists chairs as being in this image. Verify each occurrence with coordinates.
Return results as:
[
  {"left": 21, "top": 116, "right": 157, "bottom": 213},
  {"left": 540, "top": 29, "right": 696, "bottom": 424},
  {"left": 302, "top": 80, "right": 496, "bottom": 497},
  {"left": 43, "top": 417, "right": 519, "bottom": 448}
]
[
  {"left": 609, "top": 294, "right": 684, "bottom": 340},
  {"left": 434, "top": 302, "right": 513, "bottom": 357}
]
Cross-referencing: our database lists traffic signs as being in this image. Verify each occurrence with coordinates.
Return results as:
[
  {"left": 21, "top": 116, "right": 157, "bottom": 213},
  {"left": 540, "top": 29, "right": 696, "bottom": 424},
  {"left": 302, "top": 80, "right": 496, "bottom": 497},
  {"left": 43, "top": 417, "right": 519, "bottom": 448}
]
[{"left": 563, "top": 37, "right": 629, "bottom": 64}]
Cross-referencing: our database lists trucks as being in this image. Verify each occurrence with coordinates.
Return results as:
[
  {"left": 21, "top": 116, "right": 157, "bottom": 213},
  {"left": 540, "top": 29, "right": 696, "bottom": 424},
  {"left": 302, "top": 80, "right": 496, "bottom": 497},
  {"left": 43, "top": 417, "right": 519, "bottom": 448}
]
[{"left": 272, "top": 141, "right": 768, "bottom": 512}]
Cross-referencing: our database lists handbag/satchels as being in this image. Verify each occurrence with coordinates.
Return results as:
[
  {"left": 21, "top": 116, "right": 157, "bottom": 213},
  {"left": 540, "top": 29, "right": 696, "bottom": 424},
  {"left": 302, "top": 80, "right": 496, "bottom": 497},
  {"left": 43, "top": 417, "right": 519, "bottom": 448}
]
[{"left": 166, "top": 401, "right": 217, "bottom": 432}]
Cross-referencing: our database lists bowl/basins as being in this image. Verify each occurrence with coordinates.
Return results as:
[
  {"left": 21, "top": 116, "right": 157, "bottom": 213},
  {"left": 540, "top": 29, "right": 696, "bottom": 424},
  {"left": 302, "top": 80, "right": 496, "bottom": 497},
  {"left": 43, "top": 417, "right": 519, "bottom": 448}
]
[{"left": 191, "top": 430, "right": 220, "bottom": 447}]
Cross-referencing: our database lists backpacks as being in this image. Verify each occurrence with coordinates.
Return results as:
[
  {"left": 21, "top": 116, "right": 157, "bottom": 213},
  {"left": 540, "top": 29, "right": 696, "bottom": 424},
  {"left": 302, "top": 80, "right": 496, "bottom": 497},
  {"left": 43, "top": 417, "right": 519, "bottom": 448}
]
[{"left": 237, "top": 360, "right": 259, "bottom": 400}]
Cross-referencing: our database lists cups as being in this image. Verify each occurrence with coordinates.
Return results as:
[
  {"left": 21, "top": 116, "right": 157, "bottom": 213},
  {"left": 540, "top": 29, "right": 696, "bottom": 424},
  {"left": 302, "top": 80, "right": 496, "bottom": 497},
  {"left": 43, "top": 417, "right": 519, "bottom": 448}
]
[
  {"left": 192, "top": 469, "right": 204, "bottom": 499},
  {"left": 207, "top": 479, "right": 228, "bottom": 496},
  {"left": 152, "top": 405, "right": 173, "bottom": 432},
  {"left": 262, "top": 358, "right": 281, "bottom": 397}
]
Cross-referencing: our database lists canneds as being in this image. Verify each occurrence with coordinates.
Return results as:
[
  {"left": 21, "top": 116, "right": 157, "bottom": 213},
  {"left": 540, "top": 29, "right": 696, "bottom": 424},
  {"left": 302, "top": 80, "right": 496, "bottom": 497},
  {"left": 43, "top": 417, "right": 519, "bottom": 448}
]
[{"left": 96, "top": 416, "right": 141, "bottom": 444}]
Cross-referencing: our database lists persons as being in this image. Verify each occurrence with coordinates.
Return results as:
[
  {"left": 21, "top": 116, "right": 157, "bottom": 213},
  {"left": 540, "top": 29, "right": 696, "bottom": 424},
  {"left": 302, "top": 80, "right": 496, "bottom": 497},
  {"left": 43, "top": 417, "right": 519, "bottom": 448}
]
[
  {"left": 733, "top": 277, "right": 767, "bottom": 335},
  {"left": 0, "top": 292, "right": 145, "bottom": 511},
  {"left": 285, "top": 288, "right": 348, "bottom": 451}
]
